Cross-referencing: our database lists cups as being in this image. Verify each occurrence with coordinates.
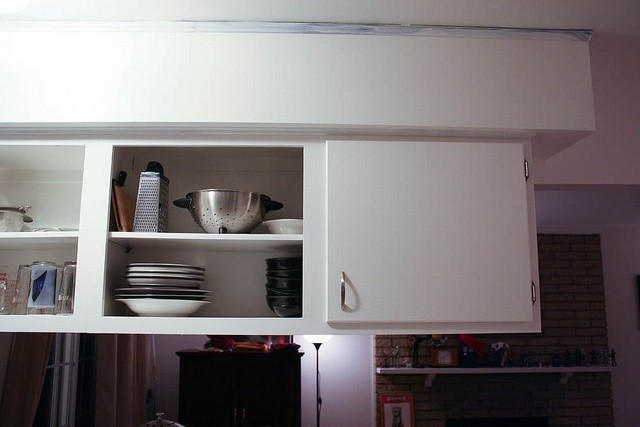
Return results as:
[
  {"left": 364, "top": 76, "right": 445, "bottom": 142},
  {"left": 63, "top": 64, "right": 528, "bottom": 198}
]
[
  {"left": 61, "top": 262, "right": 76, "bottom": 315},
  {"left": 54, "top": 265, "right": 62, "bottom": 314},
  {"left": 0, "top": 265, "right": 10, "bottom": 314},
  {"left": 29, "top": 261, "right": 56, "bottom": 314},
  {"left": 13, "top": 265, "right": 28, "bottom": 314}
]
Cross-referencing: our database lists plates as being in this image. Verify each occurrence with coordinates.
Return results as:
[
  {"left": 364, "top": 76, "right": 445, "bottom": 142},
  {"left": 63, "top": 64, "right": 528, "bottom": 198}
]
[{"left": 113, "top": 261, "right": 211, "bottom": 317}]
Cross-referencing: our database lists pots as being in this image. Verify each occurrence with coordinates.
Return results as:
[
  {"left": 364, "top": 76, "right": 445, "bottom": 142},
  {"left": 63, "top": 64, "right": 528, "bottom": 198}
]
[
  {"left": 0, "top": 206, "right": 33, "bottom": 232},
  {"left": 173, "top": 189, "right": 283, "bottom": 234}
]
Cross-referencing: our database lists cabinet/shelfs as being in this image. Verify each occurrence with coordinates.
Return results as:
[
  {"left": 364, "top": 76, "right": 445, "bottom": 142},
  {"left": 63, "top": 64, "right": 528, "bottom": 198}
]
[
  {"left": 1, "top": 140, "right": 325, "bottom": 336},
  {"left": 176, "top": 348, "right": 300, "bottom": 427},
  {"left": 324, "top": 134, "right": 544, "bottom": 334}
]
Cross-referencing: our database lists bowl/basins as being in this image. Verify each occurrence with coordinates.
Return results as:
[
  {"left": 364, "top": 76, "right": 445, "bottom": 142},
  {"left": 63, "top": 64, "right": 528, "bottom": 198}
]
[
  {"left": 263, "top": 218, "right": 303, "bottom": 233},
  {"left": 265, "top": 254, "right": 301, "bottom": 317}
]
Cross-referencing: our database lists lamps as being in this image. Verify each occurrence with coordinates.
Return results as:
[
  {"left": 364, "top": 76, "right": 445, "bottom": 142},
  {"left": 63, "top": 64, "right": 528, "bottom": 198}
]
[{"left": 305, "top": 335, "right": 332, "bottom": 427}]
